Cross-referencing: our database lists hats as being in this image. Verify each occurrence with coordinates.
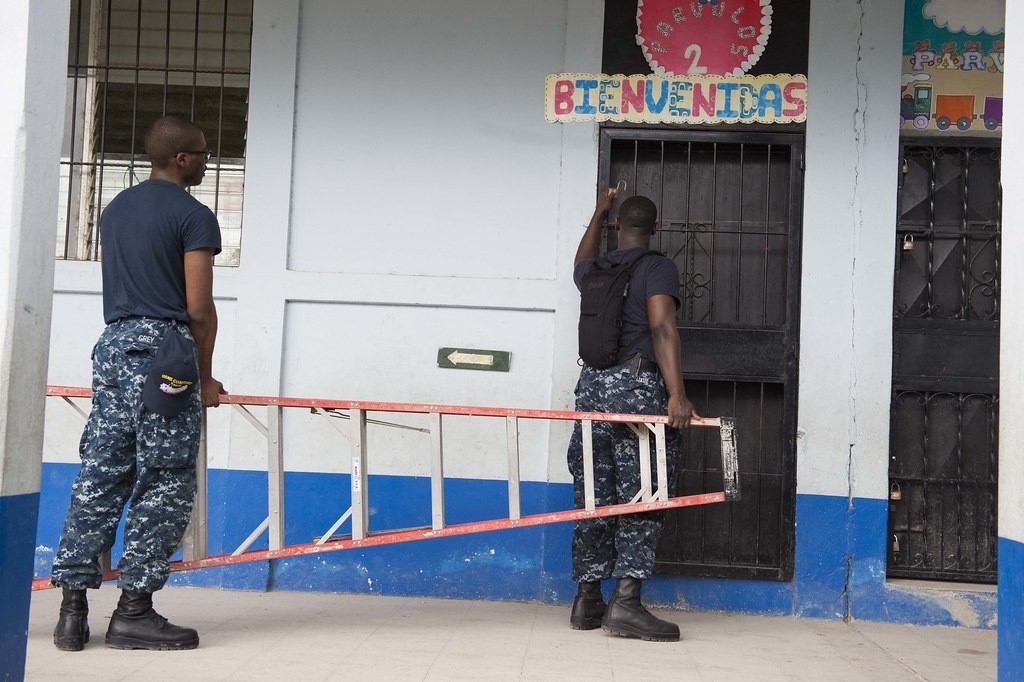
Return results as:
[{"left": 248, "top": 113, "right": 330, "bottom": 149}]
[{"left": 142, "top": 329, "right": 198, "bottom": 417}]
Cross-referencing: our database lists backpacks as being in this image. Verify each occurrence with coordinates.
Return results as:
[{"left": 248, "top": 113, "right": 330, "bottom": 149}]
[{"left": 578, "top": 250, "right": 665, "bottom": 369}]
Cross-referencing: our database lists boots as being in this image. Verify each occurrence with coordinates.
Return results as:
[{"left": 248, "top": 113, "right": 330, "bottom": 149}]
[
  {"left": 105, "top": 588, "right": 199, "bottom": 651},
  {"left": 601, "top": 576, "right": 680, "bottom": 642},
  {"left": 571, "top": 579, "right": 606, "bottom": 630},
  {"left": 54, "top": 588, "right": 90, "bottom": 651}
]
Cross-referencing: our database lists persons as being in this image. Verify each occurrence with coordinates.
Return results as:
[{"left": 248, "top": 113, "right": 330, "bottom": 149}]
[
  {"left": 568, "top": 185, "right": 703, "bottom": 640},
  {"left": 50, "top": 114, "right": 228, "bottom": 654}
]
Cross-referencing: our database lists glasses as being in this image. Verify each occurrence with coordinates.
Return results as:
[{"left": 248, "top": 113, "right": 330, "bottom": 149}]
[{"left": 174, "top": 150, "right": 213, "bottom": 160}]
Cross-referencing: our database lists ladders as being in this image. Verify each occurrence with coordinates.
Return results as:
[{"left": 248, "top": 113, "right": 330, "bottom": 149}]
[{"left": 30, "top": 385, "right": 744, "bottom": 591}]
